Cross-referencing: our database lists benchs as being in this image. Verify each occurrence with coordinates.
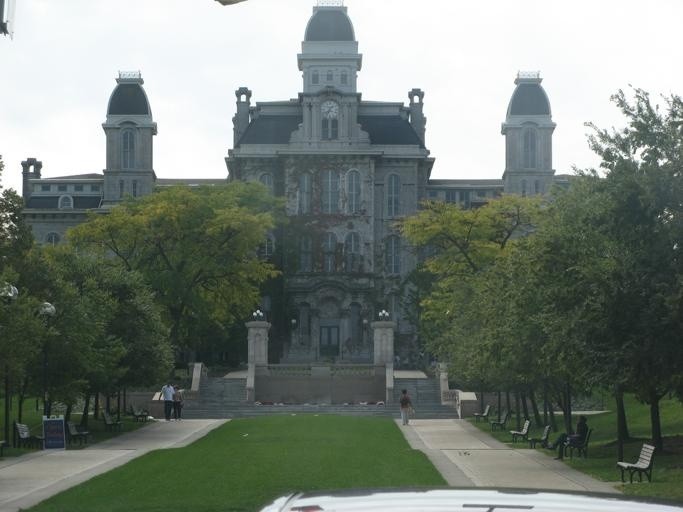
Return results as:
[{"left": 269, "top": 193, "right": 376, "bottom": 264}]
[
  {"left": 16, "top": 405, "right": 147, "bottom": 449},
  {"left": 472, "top": 404, "right": 657, "bottom": 483}
]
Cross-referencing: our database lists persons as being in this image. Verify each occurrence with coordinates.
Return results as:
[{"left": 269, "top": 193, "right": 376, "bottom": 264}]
[
  {"left": 398, "top": 388, "right": 414, "bottom": 426},
  {"left": 157, "top": 379, "right": 175, "bottom": 422},
  {"left": 172, "top": 385, "right": 182, "bottom": 421},
  {"left": 545, "top": 413, "right": 589, "bottom": 461}
]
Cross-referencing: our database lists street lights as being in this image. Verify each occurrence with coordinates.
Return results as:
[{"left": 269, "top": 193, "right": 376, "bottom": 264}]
[
  {"left": 39, "top": 301, "right": 56, "bottom": 414},
  {"left": 251, "top": 309, "right": 263, "bottom": 320},
  {"left": 377, "top": 309, "right": 390, "bottom": 321},
  {"left": 0, "top": 280, "right": 18, "bottom": 447}
]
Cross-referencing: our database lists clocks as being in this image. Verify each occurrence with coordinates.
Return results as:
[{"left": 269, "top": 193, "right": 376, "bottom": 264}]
[{"left": 320, "top": 100, "right": 340, "bottom": 120}]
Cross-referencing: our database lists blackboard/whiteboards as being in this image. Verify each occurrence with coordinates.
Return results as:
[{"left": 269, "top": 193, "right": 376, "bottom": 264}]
[{"left": 42, "top": 414, "right": 66, "bottom": 451}]
